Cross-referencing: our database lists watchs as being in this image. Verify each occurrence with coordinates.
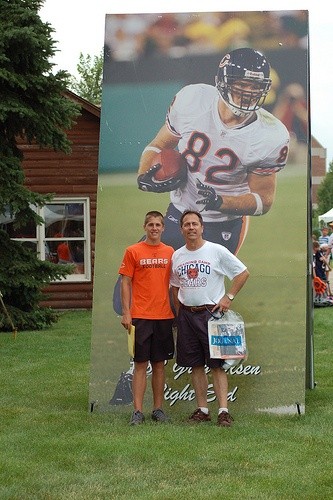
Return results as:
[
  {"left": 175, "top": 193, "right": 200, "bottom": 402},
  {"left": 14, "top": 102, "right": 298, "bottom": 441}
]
[{"left": 226, "top": 292, "right": 235, "bottom": 301}]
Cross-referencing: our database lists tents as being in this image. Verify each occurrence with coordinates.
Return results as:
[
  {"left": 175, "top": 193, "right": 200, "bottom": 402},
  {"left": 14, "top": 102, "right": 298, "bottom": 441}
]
[{"left": 319, "top": 208, "right": 333, "bottom": 227}]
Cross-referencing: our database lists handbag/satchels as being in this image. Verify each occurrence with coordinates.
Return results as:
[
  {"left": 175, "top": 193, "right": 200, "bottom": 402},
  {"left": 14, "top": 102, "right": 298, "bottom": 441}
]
[
  {"left": 110, "top": 372, "right": 132, "bottom": 405},
  {"left": 208, "top": 309, "right": 249, "bottom": 361}
]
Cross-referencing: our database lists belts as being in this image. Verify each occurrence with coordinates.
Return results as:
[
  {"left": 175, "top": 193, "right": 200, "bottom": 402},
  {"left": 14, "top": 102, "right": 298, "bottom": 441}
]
[{"left": 181, "top": 305, "right": 216, "bottom": 312}]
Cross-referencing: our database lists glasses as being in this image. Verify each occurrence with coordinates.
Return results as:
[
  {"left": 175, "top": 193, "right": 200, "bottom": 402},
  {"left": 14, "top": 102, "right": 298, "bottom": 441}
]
[{"left": 204, "top": 300, "right": 224, "bottom": 319}]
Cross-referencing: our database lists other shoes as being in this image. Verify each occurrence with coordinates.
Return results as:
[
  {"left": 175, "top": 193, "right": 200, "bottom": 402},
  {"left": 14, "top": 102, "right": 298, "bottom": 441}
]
[{"left": 124, "top": 360, "right": 152, "bottom": 382}]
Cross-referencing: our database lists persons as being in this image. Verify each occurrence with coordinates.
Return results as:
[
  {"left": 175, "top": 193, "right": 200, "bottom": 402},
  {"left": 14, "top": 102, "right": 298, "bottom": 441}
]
[
  {"left": 120, "top": 211, "right": 177, "bottom": 424},
  {"left": 171, "top": 209, "right": 250, "bottom": 426},
  {"left": 313, "top": 224, "right": 333, "bottom": 295},
  {"left": 107, "top": 10, "right": 308, "bottom": 135},
  {"left": 108, "top": 47, "right": 290, "bottom": 404},
  {"left": 6, "top": 216, "right": 83, "bottom": 263}
]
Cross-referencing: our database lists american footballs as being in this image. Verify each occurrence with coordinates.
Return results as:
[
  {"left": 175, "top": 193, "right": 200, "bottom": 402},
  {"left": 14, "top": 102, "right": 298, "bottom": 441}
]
[{"left": 150, "top": 149, "right": 188, "bottom": 184}]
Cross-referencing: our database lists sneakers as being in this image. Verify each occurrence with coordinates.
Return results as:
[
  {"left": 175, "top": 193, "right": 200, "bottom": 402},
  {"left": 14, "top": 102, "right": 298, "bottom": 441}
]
[
  {"left": 128, "top": 410, "right": 146, "bottom": 426},
  {"left": 188, "top": 409, "right": 211, "bottom": 422},
  {"left": 217, "top": 411, "right": 234, "bottom": 426},
  {"left": 152, "top": 409, "right": 166, "bottom": 421}
]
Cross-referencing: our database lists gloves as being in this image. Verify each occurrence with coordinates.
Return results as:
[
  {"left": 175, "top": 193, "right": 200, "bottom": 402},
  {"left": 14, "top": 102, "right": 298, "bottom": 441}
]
[
  {"left": 137, "top": 164, "right": 183, "bottom": 194},
  {"left": 193, "top": 177, "right": 223, "bottom": 213}
]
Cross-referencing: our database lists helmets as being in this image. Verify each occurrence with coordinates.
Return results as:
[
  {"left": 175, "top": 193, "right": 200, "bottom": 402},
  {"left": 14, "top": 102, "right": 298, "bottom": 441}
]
[{"left": 216, "top": 48, "right": 270, "bottom": 101}]
